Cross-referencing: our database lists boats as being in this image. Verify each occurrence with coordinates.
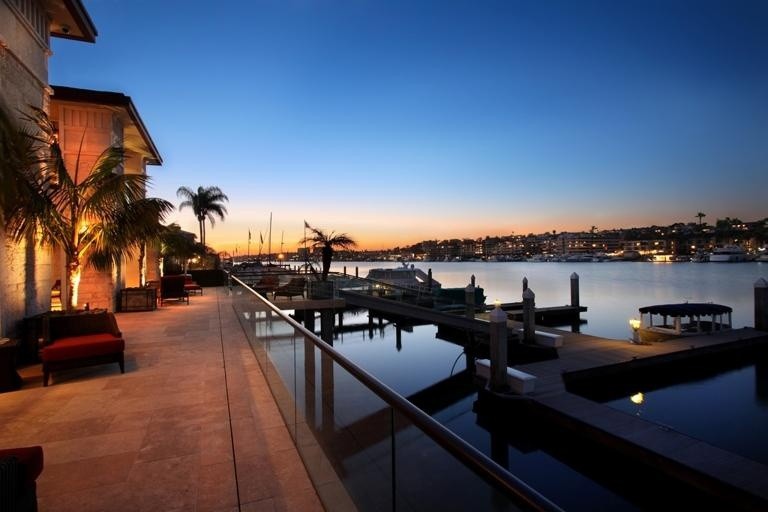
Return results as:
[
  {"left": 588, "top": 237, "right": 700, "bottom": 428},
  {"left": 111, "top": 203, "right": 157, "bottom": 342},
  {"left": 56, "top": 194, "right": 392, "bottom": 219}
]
[
  {"left": 524, "top": 253, "right": 602, "bottom": 262},
  {"left": 336, "top": 264, "right": 441, "bottom": 299},
  {"left": 629, "top": 300, "right": 732, "bottom": 347},
  {"left": 688, "top": 246, "right": 744, "bottom": 262}
]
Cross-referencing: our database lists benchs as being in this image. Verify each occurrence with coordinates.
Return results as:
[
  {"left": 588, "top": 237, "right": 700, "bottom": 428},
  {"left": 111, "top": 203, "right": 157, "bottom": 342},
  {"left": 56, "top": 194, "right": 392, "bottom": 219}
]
[
  {"left": 158, "top": 276, "right": 189, "bottom": 307},
  {"left": 144, "top": 281, "right": 161, "bottom": 304},
  {"left": 38, "top": 308, "right": 125, "bottom": 387},
  {"left": 274, "top": 277, "right": 305, "bottom": 301},
  {"left": 178, "top": 275, "right": 202, "bottom": 296},
  {"left": 251, "top": 277, "right": 280, "bottom": 297}
]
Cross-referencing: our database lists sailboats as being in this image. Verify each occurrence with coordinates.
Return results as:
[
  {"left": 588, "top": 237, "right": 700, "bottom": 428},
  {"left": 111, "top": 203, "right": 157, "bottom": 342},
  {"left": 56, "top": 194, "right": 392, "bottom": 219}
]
[{"left": 221, "top": 209, "right": 337, "bottom": 285}]
